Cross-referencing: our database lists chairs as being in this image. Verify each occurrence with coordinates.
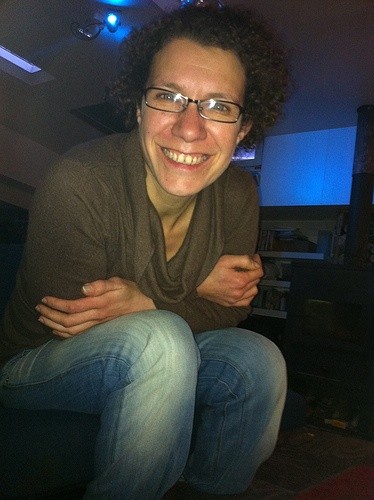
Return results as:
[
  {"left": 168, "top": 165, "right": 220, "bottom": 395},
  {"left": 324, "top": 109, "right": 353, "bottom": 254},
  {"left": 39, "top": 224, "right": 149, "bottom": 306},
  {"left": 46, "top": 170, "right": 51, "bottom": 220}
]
[{"left": 1, "top": 243, "right": 98, "bottom": 499}]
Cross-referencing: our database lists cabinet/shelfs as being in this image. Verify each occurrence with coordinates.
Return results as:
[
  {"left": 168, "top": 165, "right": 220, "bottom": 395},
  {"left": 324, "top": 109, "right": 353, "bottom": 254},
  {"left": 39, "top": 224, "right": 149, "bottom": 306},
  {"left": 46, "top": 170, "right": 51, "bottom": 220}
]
[
  {"left": 284, "top": 262, "right": 374, "bottom": 361},
  {"left": 251, "top": 251, "right": 324, "bottom": 319}
]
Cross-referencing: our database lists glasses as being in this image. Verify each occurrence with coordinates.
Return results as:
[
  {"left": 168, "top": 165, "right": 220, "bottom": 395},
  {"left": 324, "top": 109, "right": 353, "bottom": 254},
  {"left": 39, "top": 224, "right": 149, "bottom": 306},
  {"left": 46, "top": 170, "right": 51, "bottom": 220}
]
[{"left": 143, "top": 87, "right": 244, "bottom": 123}]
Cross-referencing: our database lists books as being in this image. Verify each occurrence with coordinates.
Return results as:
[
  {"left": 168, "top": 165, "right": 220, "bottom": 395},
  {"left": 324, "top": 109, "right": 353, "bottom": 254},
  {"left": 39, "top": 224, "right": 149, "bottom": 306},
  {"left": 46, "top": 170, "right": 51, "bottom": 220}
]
[
  {"left": 256, "top": 229, "right": 295, "bottom": 251},
  {"left": 252, "top": 287, "right": 288, "bottom": 311}
]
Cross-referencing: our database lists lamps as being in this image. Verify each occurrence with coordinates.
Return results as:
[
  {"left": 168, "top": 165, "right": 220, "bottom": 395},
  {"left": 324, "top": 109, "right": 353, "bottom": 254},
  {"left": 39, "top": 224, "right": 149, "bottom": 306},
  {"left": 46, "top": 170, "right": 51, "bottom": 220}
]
[{"left": 104, "top": 10, "right": 122, "bottom": 33}]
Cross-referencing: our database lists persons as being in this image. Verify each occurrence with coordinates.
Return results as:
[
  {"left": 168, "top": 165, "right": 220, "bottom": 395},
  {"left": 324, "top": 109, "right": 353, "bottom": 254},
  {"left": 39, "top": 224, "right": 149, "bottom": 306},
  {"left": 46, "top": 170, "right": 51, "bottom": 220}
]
[{"left": 0, "top": 0, "right": 289, "bottom": 500}]
